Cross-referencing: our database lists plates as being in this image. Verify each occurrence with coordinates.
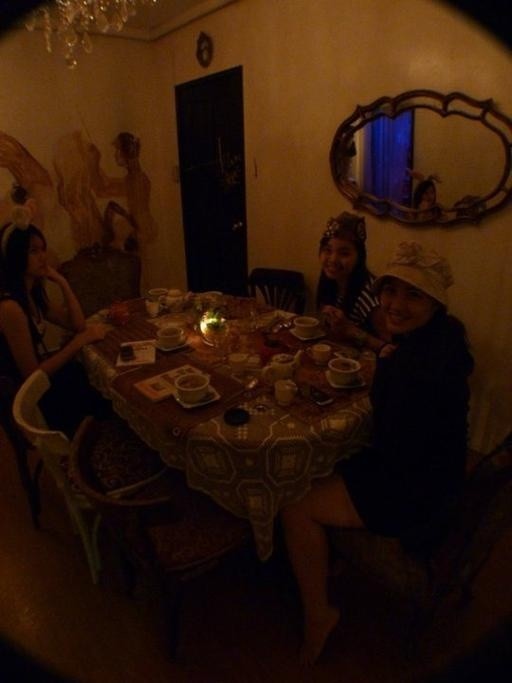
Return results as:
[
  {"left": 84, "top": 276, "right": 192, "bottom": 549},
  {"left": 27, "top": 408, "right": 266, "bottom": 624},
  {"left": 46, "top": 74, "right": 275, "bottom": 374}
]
[
  {"left": 155, "top": 336, "right": 193, "bottom": 351},
  {"left": 173, "top": 386, "right": 221, "bottom": 408},
  {"left": 325, "top": 371, "right": 366, "bottom": 388},
  {"left": 289, "top": 328, "right": 327, "bottom": 340}
]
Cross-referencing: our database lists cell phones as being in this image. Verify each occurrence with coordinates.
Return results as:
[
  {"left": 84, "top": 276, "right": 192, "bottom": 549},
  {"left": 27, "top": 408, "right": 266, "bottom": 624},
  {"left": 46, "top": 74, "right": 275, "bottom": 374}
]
[
  {"left": 118, "top": 343, "right": 134, "bottom": 361},
  {"left": 306, "top": 387, "right": 334, "bottom": 407}
]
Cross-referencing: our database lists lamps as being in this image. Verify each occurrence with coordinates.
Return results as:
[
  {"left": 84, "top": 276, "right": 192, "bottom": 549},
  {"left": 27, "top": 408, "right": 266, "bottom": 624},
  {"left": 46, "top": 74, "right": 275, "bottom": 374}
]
[{"left": 25, "top": 0, "right": 159, "bottom": 70}]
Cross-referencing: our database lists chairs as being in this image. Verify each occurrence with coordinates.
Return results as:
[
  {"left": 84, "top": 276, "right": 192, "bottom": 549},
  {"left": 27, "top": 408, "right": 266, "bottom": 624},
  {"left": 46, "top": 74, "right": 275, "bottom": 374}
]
[
  {"left": 248, "top": 267, "right": 309, "bottom": 316},
  {"left": 349, "top": 432, "right": 512, "bottom": 663},
  {"left": 58, "top": 241, "right": 141, "bottom": 319},
  {"left": 70, "top": 416, "right": 251, "bottom": 667},
  {"left": 12, "top": 368, "right": 114, "bottom": 586}
]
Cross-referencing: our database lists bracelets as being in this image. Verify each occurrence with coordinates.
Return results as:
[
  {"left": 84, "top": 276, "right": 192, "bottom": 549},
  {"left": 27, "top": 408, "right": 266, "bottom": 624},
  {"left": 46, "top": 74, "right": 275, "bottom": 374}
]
[{"left": 352, "top": 329, "right": 367, "bottom": 348}]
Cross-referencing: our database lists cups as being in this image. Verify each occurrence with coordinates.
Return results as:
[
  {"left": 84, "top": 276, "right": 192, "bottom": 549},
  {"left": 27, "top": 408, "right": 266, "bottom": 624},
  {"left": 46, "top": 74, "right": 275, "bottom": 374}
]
[
  {"left": 274, "top": 380, "right": 298, "bottom": 406},
  {"left": 157, "top": 326, "right": 184, "bottom": 348},
  {"left": 145, "top": 300, "right": 164, "bottom": 318},
  {"left": 295, "top": 317, "right": 319, "bottom": 337},
  {"left": 329, "top": 358, "right": 361, "bottom": 385},
  {"left": 306, "top": 345, "right": 331, "bottom": 365},
  {"left": 149, "top": 288, "right": 168, "bottom": 300},
  {"left": 175, "top": 374, "right": 210, "bottom": 403}
]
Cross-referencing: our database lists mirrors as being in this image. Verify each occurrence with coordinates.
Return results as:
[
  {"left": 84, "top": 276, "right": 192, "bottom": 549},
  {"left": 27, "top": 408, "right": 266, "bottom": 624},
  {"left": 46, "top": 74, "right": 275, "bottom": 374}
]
[{"left": 328, "top": 87, "right": 512, "bottom": 228}]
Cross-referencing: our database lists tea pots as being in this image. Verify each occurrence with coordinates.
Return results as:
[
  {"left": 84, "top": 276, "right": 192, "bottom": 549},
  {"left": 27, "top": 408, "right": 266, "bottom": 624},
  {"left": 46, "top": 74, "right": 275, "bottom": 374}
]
[
  {"left": 263, "top": 350, "right": 305, "bottom": 386},
  {"left": 158, "top": 286, "right": 192, "bottom": 314}
]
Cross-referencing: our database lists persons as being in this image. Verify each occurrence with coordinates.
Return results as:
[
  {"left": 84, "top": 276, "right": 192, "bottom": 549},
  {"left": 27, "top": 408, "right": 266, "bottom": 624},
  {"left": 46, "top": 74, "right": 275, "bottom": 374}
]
[
  {"left": 406, "top": 169, "right": 442, "bottom": 208},
  {"left": 314, "top": 211, "right": 397, "bottom": 353},
  {"left": 0, "top": 197, "right": 106, "bottom": 435},
  {"left": 279, "top": 239, "right": 474, "bottom": 669}
]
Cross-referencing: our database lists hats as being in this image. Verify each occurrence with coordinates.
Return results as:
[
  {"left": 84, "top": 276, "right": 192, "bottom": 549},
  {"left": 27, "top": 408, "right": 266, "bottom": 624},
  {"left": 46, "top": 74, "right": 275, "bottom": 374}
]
[
  {"left": 323, "top": 211, "right": 367, "bottom": 242},
  {"left": 369, "top": 243, "right": 452, "bottom": 308}
]
